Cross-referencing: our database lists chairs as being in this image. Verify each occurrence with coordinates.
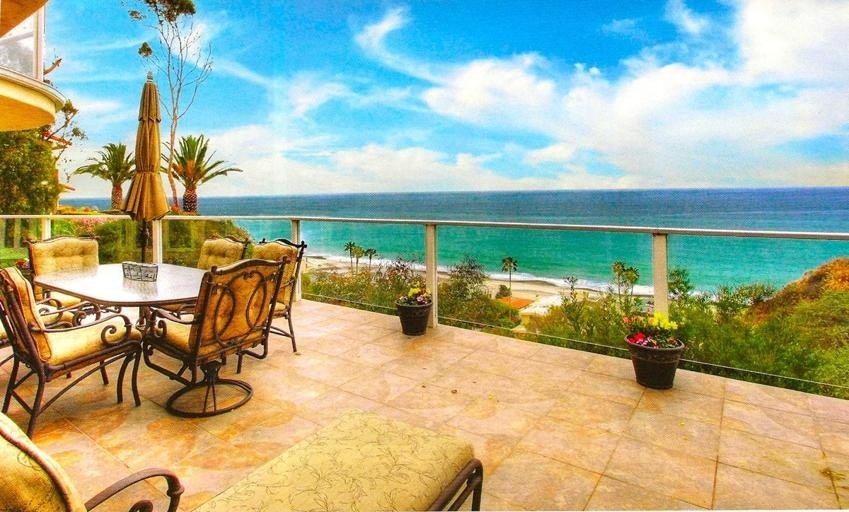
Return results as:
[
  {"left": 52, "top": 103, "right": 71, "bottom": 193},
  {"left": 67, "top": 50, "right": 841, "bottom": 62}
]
[
  {"left": 0, "top": 288, "right": 85, "bottom": 347},
  {"left": 0, "top": 265, "right": 143, "bottom": 439},
  {"left": 0, "top": 407, "right": 484, "bottom": 511},
  {"left": 252, "top": 238, "right": 306, "bottom": 352},
  {"left": 22, "top": 235, "right": 121, "bottom": 314},
  {"left": 155, "top": 234, "right": 251, "bottom": 320},
  {"left": 138, "top": 255, "right": 288, "bottom": 418}
]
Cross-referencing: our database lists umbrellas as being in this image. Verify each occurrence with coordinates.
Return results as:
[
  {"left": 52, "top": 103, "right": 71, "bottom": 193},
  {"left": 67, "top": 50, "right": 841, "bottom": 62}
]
[{"left": 119, "top": 70, "right": 171, "bottom": 328}]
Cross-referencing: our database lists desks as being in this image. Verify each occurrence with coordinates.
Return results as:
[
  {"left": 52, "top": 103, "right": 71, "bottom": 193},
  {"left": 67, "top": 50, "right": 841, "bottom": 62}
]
[{"left": 33, "top": 262, "right": 210, "bottom": 328}]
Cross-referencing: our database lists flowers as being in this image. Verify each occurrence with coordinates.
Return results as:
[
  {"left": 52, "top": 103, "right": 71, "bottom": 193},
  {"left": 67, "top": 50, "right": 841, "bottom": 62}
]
[
  {"left": 16, "top": 257, "right": 32, "bottom": 270},
  {"left": 399, "top": 279, "right": 431, "bottom": 306},
  {"left": 624, "top": 301, "right": 679, "bottom": 347}
]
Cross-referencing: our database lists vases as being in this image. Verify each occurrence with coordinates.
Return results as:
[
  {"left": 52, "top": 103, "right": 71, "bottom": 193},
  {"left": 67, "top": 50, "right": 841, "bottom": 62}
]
[
  {"left": 19, "top": 268, "right": 33, "bottom": 286},
  {"left": 395, "top": 298, "right": 432, "bottom": 336},
  {"left": 625, "top": 333, "right": 684, "bottom": 389}
]
